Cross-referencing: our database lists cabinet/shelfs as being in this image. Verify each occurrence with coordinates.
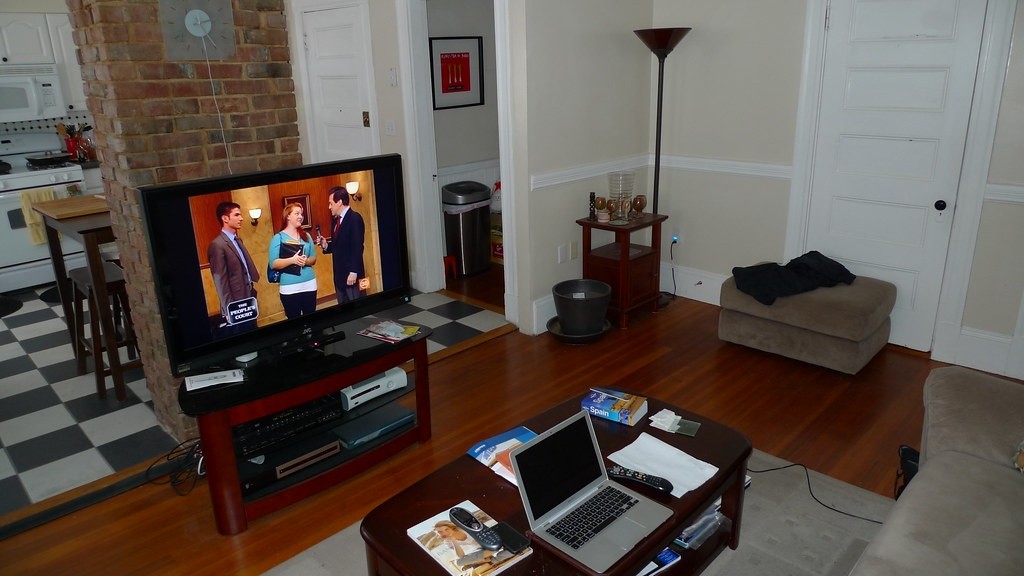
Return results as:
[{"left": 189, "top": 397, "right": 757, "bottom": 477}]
[
  {"left": 576, "top": 210, "right": 669, "bottom": 330},
  {"left": 178, "top": 317, "right": 433, "bottom": 536},
  {"left": 83, "top": 170, "right": 120, "bottom": 260},
  {"left": 0, "top": 11, "right": 87, "bottom": 124}
]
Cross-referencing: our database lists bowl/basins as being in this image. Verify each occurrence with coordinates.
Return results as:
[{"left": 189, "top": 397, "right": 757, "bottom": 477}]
[
  {"left": 552, "top": 279, "right": 612, "bottom": 334},
  {"left": 547, "top": 316, "right": 610, "bottom": 345}
]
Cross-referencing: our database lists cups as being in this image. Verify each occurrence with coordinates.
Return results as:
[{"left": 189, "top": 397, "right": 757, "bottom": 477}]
[{"left": 65, "top": 138, "right": 82, "bottom": 159}]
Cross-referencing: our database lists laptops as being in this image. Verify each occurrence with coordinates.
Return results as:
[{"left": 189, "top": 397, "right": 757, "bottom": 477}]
[{"left": 509, "top": 410, "right": 674, "bottom": 573}]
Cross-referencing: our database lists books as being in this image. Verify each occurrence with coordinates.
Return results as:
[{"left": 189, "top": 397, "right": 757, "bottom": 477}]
[
  {"left": 636, "top": 546, "right": 682, "bottom": 576},
  {"left": 356, "top": 318, "right": 421, "bottom": 344},
  {"left": 674, "top": 474, "right": 752, "bottom": 548},
  {"left": 407, "top": 499, "right": 533, "bottom": 576},
  {"left": 581, "top": 386, "right": 648, "bottom": 427}
]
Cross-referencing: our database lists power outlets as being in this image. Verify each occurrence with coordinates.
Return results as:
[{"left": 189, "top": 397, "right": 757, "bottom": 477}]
[{"left": 670, "top": 228, "right": 680, "bottom": 246}]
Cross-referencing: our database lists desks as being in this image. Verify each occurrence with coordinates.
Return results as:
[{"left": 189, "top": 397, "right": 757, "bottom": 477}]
[{"left": 32, "top": 194, "right": 144, "bottom": 403}]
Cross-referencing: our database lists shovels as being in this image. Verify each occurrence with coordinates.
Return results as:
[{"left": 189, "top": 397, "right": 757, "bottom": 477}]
[{"left": 57, "top": 122, "right": 69, "bottom": 138}]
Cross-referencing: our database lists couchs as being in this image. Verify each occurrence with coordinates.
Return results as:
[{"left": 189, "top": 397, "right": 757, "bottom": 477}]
[{"left": 848, "top": 365, "right": 1024, "bottom": 576}]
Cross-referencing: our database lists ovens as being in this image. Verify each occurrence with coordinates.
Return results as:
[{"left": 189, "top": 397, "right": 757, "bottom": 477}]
[{"left": 0, "top": 173, "right": 88, "bottom": 293}]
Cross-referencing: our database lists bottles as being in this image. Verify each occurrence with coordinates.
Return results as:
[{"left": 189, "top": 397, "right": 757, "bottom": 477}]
[{"left": 75, "top": 139, "right": 97, "bottom": 162}]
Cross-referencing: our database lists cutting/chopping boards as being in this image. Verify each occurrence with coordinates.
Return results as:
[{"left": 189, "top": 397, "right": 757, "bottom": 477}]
[{"left": 31, "top": 193, "right": 110, "bottom": 219}]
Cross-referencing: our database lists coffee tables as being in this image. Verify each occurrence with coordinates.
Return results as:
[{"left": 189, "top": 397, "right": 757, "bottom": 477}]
[{"left": 359, "top": 385, "right": 753, "bottom": 576}]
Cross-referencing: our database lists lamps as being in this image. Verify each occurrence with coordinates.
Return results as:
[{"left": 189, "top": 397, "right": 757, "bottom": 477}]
[{"left": 633, "top": 27, "right": 693, "bottom": 309}]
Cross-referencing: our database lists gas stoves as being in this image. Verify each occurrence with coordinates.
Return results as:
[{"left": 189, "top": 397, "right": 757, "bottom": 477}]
[{"left": 0, "top": 135, "right": 84, "bottom": 192}]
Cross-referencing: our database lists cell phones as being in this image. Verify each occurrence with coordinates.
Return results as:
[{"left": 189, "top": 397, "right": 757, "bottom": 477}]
[{"left": 490, "top": 521, "right": 530, "bottom": 554}]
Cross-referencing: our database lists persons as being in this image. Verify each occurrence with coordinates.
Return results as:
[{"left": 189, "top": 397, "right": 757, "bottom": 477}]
[
  {"left": 318, "top": 186, "right": 367, "bottom": 304},
  {"left": 207, "top": 202, "right": 260, "bottom": 318},
  {"left": 269, "top": 203, "right": 317, "bottom": 319}
]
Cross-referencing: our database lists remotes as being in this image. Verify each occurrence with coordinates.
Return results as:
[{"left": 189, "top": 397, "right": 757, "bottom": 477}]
[
  {"left": 449, "top": 507, "right": 503, "bottom": 551},
  {"left": 605, "top": 465, "right": 673, "bottom": 494}
]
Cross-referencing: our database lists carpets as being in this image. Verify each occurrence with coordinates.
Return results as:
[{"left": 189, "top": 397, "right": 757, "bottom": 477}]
[
  {"left": 261, "top": 448, "right": 898, "bottom": 576},
  {"left": 39, "top": 283, "right": 87, "bottom": 304},
  {"left": 0, "top": 295, "right": 24, "bottom": 318}
]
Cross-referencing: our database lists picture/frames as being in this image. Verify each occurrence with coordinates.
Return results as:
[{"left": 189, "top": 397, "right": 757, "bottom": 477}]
[{"left": 428, "top": 36, "right": 485, "bottom": 111}]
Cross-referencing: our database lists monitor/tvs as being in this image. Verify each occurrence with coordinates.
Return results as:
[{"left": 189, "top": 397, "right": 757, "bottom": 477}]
[{"left": 136, "top": 152, "right": 412, "bottom": 378}]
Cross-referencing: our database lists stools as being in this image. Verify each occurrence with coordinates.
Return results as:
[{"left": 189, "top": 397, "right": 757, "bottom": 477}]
[
  {"left": 717, "top": 275, "right": 896, "bottom": 375},
  {"left": 68, "top": 251, "right": 142, "bottom": 402}
]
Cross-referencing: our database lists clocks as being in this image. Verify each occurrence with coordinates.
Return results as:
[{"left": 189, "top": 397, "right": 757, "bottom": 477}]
[{"left": 159, "top": 0, "right": 236, "bottom": 60}]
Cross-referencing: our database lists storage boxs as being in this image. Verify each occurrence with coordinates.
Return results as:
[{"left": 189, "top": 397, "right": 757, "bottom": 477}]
[{"left": 491, "top": 214, "right": 503, "bottom": 265}]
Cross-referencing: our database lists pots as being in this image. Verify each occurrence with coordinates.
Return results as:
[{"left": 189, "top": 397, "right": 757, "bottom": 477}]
[{"left": 25, "top": 151, "right": 74, "bottom": 166}]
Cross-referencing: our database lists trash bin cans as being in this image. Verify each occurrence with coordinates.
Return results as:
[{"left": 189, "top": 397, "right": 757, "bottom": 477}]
[{"left": 440, "top": 179, "right": 492, "bottom": 278}]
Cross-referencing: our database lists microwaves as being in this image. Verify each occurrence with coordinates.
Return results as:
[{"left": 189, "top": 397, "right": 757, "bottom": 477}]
[{"left": 0, "top": 64, "right": 67, "bottom": 124}]
